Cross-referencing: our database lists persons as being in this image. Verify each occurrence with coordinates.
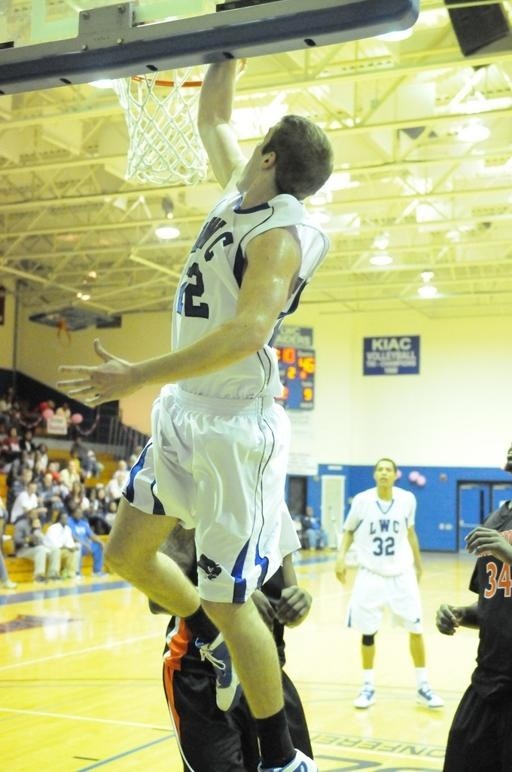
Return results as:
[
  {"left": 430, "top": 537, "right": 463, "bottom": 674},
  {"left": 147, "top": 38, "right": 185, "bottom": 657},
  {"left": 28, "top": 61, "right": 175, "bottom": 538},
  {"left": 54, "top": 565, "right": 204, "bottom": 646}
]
[
  {"left": 148, "top": 522, "right": 314, "bottom": 772},
  {"left": 334, "top": 457, "right": 444, "bottom": 710},
  {"left": 55, "top": 54, "right": 335, "bottom": 771},
  {"left": 436, "top": 443, "right": 512, "bottom": 772},
  {"left": 0, "top": 386, "right": 148, "bottom": 590},
  {"left": 299, "top": 506, "right": 330, "bottom": 551}
]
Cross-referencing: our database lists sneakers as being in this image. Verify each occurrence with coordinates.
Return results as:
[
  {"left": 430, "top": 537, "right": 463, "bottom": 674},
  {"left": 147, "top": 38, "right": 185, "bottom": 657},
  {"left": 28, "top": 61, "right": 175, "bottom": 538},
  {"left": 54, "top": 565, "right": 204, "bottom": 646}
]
[
  {"left": 93, "top": 571, "right": 109, "bottom": 578},
  {"left": 5, "top": 579, "right": 18, "bottom": 589},
  {"left": 257, "top": 748, "right": 318, "bottom": 772},
  {"left": 35, "top": 573, "right": 84, "bottom": 584},
  {"left": 352, "top": 682, "right": 377, "bottom": 708},
  {"left": 194, "top": 631, "right": 244, "bottom": 713},
  {"left": 416, "top": 686, "right": 446, "bottom": 707}
]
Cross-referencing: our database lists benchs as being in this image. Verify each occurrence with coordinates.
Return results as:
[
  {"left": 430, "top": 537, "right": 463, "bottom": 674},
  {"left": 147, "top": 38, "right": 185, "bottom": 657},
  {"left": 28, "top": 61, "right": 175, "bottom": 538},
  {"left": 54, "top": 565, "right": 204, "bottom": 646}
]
[{"left": 1, "top": 448, "right": 126, "bottom": 582}]
